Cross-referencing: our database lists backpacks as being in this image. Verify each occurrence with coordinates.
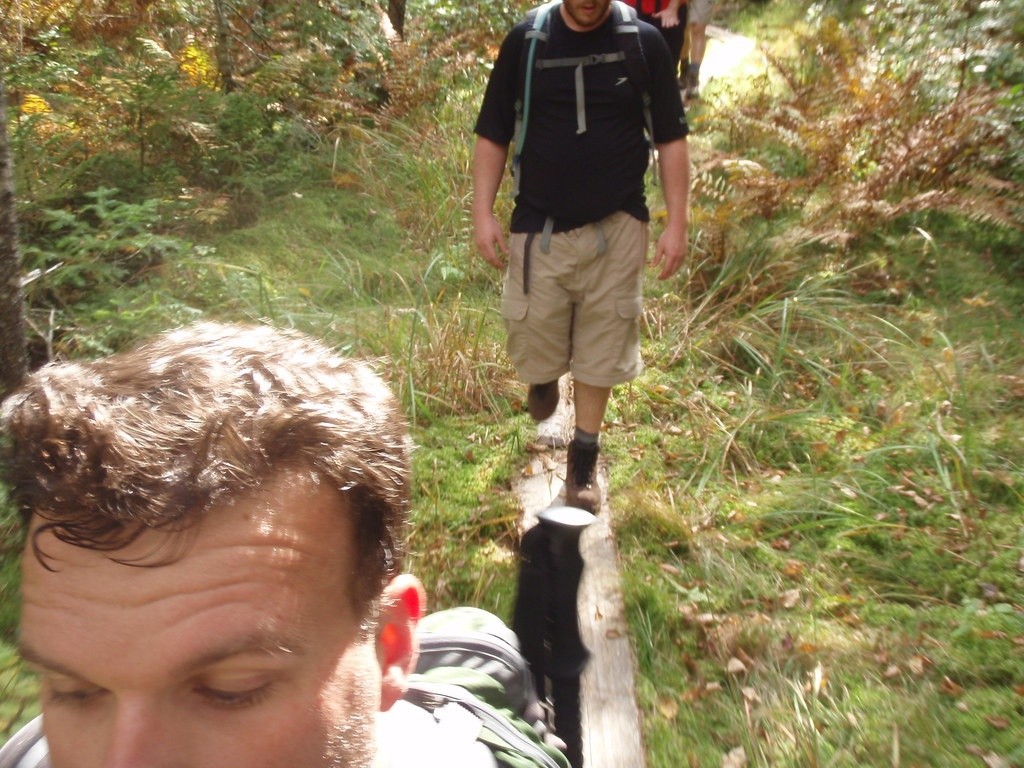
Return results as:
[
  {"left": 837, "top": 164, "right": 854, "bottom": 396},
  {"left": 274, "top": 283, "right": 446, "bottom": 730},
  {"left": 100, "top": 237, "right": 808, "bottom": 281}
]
[{"left": 389, "top": 594, "right": 575, "bottom": 768}]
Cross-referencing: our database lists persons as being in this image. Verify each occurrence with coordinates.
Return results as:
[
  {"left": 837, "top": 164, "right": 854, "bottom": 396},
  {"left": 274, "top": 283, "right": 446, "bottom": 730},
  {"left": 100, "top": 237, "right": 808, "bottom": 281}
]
[
  {"left": 472, "top": 0, "right": 692, "bottom": 518},
  {"left": 674, "top": 0, "right": 711, "bottom": 100},
  {"left": 1, "top": 319, "right": 571, "bottom": 768},
  {"left": 620, "top": 0, "right": 692, "bottom": 84}
]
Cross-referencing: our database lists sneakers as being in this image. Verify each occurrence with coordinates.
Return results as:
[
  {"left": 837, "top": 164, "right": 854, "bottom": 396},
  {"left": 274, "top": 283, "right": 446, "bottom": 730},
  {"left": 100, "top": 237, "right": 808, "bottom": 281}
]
[
  {"left": 523, "top": 375, "right": 561, "bottom": 423},
  {"left": 565, "top": 439, "right": 603, "bottom": 516},
  {"left": 685, "top": 70, "right": 701, "bottom": 100},
  {"left": 674, "top": 58, "right": 690, "bottom": 87}
]
